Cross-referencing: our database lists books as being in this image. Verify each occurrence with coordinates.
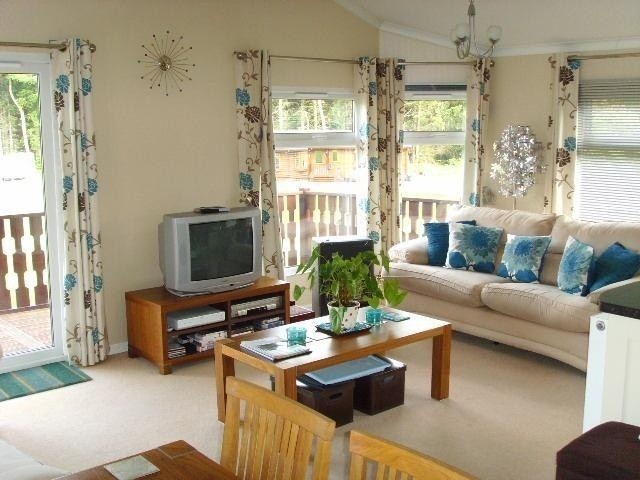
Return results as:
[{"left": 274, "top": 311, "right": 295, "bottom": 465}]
[
  {"left": 177, "top": 323, "right": 255, "bottom": 353},
  {"left": 166, "top": 342, "right": 186, "bottom": 359},
  {"left": 298, "top": 354, "right": 392, "bottom": 391},
  {"left": 240, "top": 334, "right": 312, "bottom": 363}
]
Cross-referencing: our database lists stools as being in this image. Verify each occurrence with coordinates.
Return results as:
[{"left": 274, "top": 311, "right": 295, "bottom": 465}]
[{"left": 557, "top": 421, "right": 640, "bottom": 480}]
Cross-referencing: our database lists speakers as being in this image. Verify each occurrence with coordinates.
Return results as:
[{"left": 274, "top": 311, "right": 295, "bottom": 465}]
[{"left": 311, "top": 236, "right": 373, "bottom": 317}]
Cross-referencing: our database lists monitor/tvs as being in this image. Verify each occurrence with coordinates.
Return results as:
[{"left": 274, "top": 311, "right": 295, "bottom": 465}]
[{"left": 157, "top": 207, "right": 262, "bottom": 296}]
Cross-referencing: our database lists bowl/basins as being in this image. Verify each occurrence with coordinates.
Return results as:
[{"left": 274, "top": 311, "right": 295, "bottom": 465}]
[
  {"left": 287, "top": 326, "right": 307, "bottom": 341},
  {"left": 287, "top": 341, "right": 307, "bottom": 348},
  {"left": 366, "top": 308, "right": 381, "bottom": 325}
]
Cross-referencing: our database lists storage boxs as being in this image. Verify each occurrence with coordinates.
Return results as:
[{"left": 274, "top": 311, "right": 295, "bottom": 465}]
[
  {"left": 297, "top": 374, "right": 355, "bottom": 428},
  {"left": 353, "top": 353, "right": 407, "bottom": 416}
]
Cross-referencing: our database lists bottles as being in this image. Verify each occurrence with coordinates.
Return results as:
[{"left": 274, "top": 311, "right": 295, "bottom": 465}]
[{"left": 376, "top": 274, "right": 388, "bottom": 324}]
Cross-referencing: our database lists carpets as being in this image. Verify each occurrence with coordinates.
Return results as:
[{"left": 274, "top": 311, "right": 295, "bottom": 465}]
[{"left": 0, "top": 360, "right": 93, "bottom": 402}]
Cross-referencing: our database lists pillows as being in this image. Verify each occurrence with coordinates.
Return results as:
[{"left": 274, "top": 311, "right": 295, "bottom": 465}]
[
  {"left": 495, "top": 234, "right": 552, "bottom": 284},
  {"left": 423, "top": 220, "right": 476, "bottom": 266},
  {"left": 557, "top": 235, "right": 594, "bottom": 295},
  {"left": 587, "top": 242, "right": 639, "bottom": 294},
  {"left": 443, "top": 221, "right": 504, "bottom": 273}
]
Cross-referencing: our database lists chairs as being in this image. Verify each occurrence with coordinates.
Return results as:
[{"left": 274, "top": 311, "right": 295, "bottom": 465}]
[
  {"left": 220, "top": 375, "right": 335, "bottom": 479},
  {"left": 348, "top": 429, "right": 480, "bottom": 479}
]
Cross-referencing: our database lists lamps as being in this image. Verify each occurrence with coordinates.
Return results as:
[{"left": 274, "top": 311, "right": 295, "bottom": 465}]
[{"left": 452, "top": 1, "right": 502, "bottom": 70}]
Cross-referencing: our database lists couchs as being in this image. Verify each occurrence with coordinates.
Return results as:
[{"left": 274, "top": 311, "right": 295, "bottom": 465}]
[{"left": 379, "top": 203, "right": 640, "bottom": 373}]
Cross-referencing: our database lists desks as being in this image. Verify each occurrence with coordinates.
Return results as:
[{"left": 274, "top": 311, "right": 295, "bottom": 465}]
[
  {"left": 55, "top": 441, "right": 244, "bottom": 480},
  {"left": 125, "top": 276, "right": 290, "bottom": 375}
]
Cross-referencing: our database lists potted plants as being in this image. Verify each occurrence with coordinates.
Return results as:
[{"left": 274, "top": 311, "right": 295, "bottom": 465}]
[{"left": 292, "top": 244, "right": 409, "bottom": 334}]
